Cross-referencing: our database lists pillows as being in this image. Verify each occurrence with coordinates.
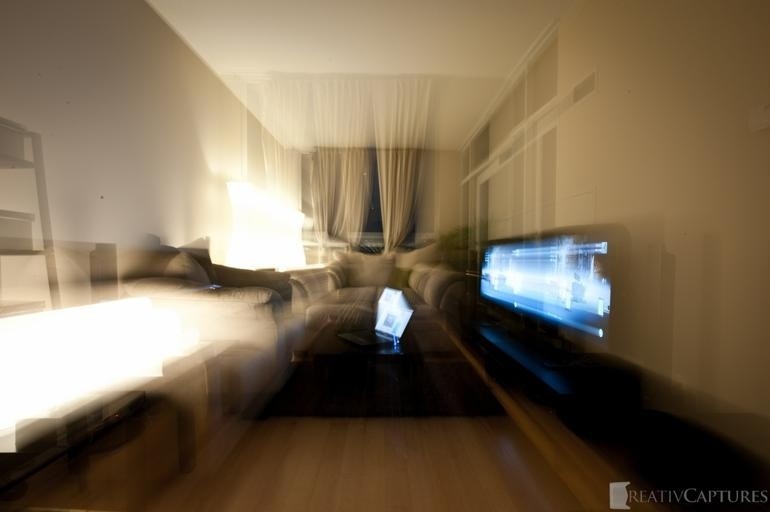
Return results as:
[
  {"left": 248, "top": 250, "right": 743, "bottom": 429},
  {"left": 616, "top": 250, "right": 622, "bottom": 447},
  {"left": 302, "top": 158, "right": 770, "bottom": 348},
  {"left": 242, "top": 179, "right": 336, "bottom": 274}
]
[{"left": 394, "top": 241, "right": 441, "bottom": 269}]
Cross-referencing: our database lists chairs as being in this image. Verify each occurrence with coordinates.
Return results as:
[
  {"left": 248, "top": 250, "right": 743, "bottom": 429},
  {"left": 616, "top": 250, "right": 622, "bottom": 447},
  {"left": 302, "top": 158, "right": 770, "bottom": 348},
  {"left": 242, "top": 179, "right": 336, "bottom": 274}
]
[{"left": 115, "top": 246, "right": 470, "bottom": 418}]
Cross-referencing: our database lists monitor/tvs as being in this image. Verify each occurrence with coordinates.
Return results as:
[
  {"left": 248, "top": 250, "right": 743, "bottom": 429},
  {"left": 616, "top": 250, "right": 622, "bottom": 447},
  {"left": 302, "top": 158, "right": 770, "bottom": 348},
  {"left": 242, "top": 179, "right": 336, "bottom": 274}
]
[{"left": 478, "top": 227, "right": 616, "bottom": 345}]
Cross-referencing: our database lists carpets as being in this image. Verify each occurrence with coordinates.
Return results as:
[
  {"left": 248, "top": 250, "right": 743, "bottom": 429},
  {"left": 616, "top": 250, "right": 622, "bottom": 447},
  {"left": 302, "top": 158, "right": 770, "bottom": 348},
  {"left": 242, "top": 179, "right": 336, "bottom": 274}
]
[{"left": 253, "top": 349, "right": 508, "bottom": 421}]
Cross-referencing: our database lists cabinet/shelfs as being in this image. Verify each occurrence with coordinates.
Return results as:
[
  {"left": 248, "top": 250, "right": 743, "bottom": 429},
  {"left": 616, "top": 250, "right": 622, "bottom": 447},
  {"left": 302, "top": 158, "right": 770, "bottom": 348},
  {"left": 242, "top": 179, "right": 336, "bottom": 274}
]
[{"left": 0, "top": 116, "right": 60, "bottom": 319}]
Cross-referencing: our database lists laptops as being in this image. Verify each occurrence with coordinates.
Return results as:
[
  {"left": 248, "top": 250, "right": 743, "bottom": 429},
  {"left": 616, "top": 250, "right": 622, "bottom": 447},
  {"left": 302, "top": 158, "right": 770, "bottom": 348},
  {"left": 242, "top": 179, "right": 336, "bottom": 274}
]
[{"left": 337, "top": 284, "right": 415, "bottom": 346}]
